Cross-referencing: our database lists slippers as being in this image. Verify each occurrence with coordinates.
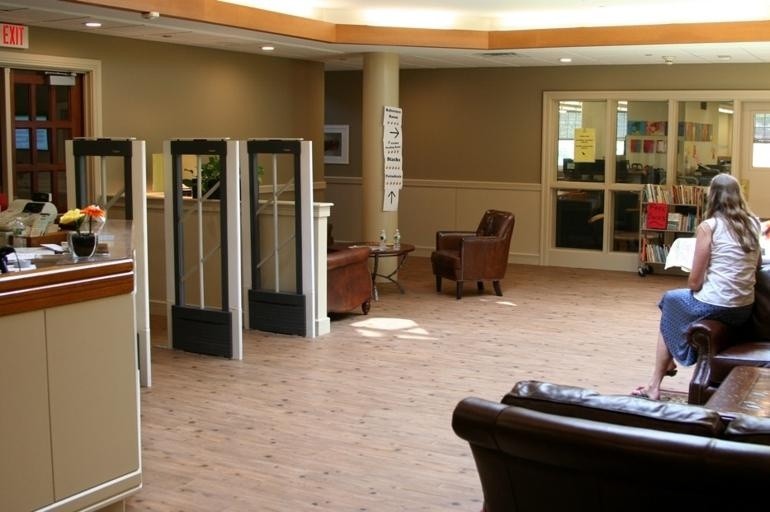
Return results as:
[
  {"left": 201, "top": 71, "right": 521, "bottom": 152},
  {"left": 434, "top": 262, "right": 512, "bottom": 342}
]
[
  {"left": 631, "top": 387, "right": 661, "bottom": 402},
  {"left": 665, "top": 369, "right": 677, "bottom": 376}
]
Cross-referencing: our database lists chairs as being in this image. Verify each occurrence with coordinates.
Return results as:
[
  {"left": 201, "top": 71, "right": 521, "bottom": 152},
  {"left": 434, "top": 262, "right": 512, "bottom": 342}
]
[
  {"left": 328, "top": 223, "right": 373, "bottom": 320},
  {"left": 430, "top": 209, "right": 516, "bottom": 300}
]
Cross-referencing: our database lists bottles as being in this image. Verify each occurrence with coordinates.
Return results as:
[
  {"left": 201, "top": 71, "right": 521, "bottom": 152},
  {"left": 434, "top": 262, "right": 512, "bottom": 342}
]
[
  {"left": 378, "top": 230, "right": 386, "bottom": 251},
  {"left": 393, "top": 229, "right": 400, "bottom": 251},
  {"left": 13, "top": 217, "right": 25, "bottom": 248}
]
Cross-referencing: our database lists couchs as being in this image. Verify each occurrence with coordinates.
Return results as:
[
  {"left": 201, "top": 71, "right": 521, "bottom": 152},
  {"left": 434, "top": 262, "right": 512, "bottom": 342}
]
[
  {"left": 686, "top": 266, "right": 770, "bottom": 406},
  {"left": 452, "top": 379, "right": 770, "bottom": 512}
]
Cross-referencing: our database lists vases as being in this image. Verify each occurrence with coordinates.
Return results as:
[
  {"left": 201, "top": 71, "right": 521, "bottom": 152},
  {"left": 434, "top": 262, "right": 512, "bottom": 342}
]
[{"left": 72, "top": 232, "right": 96, "bottom": 259}]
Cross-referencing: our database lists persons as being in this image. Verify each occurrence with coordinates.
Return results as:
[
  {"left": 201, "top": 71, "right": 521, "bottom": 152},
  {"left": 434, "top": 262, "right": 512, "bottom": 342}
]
[{"left": 631, "top": 174, "right": 763, "bottom": 401}]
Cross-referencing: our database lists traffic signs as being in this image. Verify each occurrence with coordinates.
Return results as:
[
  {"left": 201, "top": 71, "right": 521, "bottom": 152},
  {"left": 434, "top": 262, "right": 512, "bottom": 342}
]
[{"left": 382, "top": 107, "right": 403, "bottom": 189}]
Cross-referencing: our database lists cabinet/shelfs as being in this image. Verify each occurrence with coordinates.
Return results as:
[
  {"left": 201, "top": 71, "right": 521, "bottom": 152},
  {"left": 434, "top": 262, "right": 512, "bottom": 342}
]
[{"left": 1, "top": 249, "right": 144, "bottom": 512}]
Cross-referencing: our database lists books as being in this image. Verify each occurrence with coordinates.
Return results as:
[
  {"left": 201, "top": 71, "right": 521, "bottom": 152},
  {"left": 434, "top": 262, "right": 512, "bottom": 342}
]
[
  {"left": 638, "top": 183, "right": 709, "bottom": 273},
  {"left": 1, "top": 243, "right": 65, "bottom": 272}
]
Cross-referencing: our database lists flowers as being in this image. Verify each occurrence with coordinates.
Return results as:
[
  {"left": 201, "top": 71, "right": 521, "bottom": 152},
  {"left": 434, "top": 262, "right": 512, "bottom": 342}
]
[{"left": 58, "top": 204, "right": 105, "bottom": 235}]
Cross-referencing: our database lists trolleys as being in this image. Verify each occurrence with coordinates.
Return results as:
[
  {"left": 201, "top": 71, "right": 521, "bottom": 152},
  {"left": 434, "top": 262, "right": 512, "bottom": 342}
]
[{"left": 638, "top": 189, "right": 705, "bottom": 277}]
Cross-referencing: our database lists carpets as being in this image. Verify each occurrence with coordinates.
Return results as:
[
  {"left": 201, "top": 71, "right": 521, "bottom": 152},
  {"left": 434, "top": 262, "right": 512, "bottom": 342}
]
[{"left": 630, "top": 386, "right": 689, "bottom": 405}]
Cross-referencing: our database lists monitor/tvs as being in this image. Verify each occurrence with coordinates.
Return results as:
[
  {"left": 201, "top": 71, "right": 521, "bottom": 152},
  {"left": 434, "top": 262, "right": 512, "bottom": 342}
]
[{"left": 562, "top": 158, "right": 577, "bottom": 176}]
